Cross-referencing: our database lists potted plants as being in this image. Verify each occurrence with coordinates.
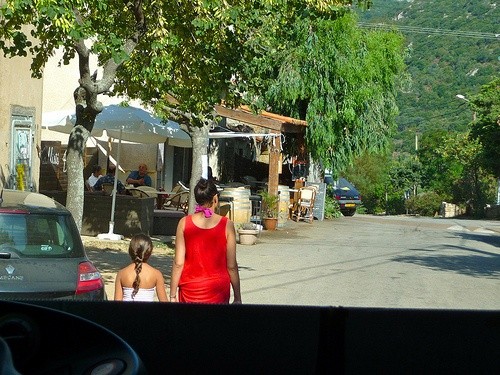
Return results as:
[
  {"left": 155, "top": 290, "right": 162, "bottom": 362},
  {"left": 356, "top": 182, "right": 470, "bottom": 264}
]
[
  {"left": 259, "top": 188, "right": 279, "bottom": 229},
  {"left": 237, "top": 222, "right": 258, "bottom": 245}
]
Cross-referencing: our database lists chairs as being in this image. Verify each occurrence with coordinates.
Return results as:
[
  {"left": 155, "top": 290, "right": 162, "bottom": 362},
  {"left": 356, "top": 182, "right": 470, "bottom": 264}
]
[
  {"left": 13, "top": 232, "right": 49, "bottom": 245},
  {"left": 101, "top": 181, "right": 189, "bottom": 211},
  {"left": 291, "top": 187, "right": 315, "bottom": 222}
]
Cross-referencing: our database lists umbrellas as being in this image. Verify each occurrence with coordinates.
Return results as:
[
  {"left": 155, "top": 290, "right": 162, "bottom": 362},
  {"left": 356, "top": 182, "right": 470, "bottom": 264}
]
[{"left": 41, "top": 102, "right": 193, "bottom": 240}]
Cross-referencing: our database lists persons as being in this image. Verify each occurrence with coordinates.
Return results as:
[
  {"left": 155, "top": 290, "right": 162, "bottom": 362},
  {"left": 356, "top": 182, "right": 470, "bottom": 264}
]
[
  {"left": 125, "top": 163, "right": 152, "bottom": 187},
  {"left": 91, "top": 165, "right": 125, "bottom": 195},
  {"left": 86, "top": 166, "right": 103, "bottom": 191},
  {"left": 112, "top": 233, "right": 169, "bottom": 303},
  {"left": 169, "top": 178, "right": 243, "bottom": 304}
]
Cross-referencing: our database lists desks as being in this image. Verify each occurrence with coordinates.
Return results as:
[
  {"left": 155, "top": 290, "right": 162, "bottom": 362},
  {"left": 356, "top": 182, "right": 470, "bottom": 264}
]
[{"left": 143, "top": 191, "right": 175, "bottom": 209}]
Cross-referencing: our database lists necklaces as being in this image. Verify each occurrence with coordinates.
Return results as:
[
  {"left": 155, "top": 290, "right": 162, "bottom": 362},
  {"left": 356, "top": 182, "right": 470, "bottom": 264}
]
[{"left": 194, "top": 204, "right": 213, "bottom": 218}]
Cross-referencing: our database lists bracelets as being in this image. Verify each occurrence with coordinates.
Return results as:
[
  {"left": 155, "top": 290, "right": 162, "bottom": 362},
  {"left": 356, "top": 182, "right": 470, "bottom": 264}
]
[{"left": 170, "top": 295, "right": 176, "bottom": 298}]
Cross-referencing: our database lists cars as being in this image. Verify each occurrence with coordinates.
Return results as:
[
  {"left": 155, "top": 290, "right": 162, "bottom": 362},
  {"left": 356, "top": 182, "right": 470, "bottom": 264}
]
[
  {"left": 0, "top": 190, "right": 107, "bottom": 300},
  {"left": 323, "top": 173, "right": 361, "bottom": 218}
]
